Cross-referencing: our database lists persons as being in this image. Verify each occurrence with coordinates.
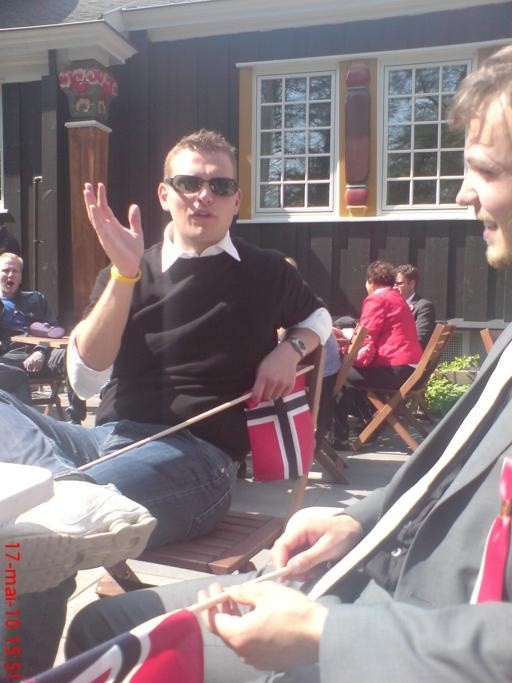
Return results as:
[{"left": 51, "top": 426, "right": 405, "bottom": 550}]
[
  {"left": 392, "top": 262, "right": 435, "bottom": 346},
  {"left": 2, "top": 250, "right": 87, "bottom": 427},
  {"left": 2, "top": 127, "right": 336, "bottom": 682},
  {"left": 331, "top": 258, "right": 422, "bottom": 455},
  {"left": 65, "top": 31, "right": 512, "bottom": 681}
]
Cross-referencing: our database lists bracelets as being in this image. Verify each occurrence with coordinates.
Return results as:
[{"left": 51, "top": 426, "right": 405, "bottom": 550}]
[{"left": 109, "top": 259, "right": 147, "bottom": 284}]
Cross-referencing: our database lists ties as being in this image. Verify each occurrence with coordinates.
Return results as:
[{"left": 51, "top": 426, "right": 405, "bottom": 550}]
[{"left": 304, "top": 338, "right": 512, "bottom": 602}]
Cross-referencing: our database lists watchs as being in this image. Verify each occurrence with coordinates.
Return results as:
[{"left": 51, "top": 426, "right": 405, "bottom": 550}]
[{"left": 280, "top": 332, "right": 311, "bottom": 358}]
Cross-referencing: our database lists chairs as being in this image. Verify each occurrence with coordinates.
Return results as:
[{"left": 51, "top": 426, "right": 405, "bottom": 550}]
[
  {"left": 349, "top": 323, "right": 455, "bottom": 453},
  {"left": 313, "top": 322, "right": 363, "bottom": 484},
  {"left": 143, "top": 344, "right": 324, "bottom": 576}
]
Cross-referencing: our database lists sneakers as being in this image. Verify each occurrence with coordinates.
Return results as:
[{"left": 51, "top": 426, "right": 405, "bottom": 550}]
[
  {"left": 0, "top": 481, "right": 158, "bottom": 596},
  {"left": 325, "top": 432, "right": 351, "bottom": 451},
  {"left": 65, "top": 407, "right": 81, "bottom": 424}
]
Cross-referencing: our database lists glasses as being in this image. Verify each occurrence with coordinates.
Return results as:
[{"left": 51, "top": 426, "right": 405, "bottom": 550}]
[{"left": 164, "top": 175, "right": 239, "bottom": 197}]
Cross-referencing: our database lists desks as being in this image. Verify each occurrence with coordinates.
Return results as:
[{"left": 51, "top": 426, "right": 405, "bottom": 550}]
[{"left": 11, "top": 335, "right": 70, "bottom": 422}]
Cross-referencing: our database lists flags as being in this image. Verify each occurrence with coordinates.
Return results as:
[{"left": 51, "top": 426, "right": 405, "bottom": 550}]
[
  {"left": 243, "top": 371, "right": 316, "bottom": 479},
  {"left": 17, "top": 606, "right": 204, "bottom": 683}
]
